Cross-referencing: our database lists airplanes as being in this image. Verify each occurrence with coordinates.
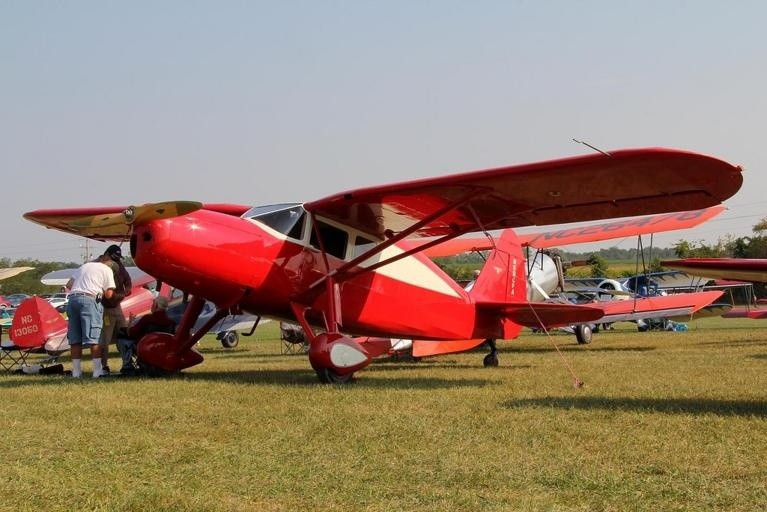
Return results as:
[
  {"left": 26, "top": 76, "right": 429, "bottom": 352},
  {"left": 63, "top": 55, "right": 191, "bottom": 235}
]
[
  {"left": 659, "top": 255, "right": 767, "bottom": 282},
  {"left": 562, "top": 271, "right": 687, "bottom": 330},
  {"left": 11, "top": 264, "right": 272, "bottom": 364},
  {"left": 23, "top": 145, "right": 745, "bottom": 381},
  {"left": 457, "top": 248, "right": 727, "bottom": 343}
]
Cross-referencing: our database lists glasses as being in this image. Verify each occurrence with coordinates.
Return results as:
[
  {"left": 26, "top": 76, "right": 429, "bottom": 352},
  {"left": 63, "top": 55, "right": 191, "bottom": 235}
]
[{"left": 112, "top": 258, "right": 120, "bottom": 261}]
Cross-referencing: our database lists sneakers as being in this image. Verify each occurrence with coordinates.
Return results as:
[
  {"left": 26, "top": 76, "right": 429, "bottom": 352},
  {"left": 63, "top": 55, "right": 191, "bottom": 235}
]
[{"left": 72, "top": 366, "right": 146, "bottom": 378}]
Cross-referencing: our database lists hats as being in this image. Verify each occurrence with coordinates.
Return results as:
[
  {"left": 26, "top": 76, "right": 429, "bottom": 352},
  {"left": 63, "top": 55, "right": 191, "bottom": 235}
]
[{"left": 108, "top": 245, "right": 123, "bottom": 259}]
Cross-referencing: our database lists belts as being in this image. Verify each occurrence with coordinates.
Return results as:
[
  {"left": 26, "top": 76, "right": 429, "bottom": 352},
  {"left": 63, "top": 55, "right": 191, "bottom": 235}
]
[{"left": 68, "top": 293, "right": 95, "bottom": 298}]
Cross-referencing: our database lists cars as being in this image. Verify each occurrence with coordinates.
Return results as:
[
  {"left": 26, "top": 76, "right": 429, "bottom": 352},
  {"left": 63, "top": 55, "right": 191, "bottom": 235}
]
[{"left": 0, "top": 292, "right": 70, "bottom": 312}]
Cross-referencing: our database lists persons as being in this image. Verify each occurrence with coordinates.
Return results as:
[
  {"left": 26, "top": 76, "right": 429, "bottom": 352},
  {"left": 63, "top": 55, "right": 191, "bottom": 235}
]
[
  {"left": 95, "top": 244, "right": 132, "bottom": 375},
  {"left": 64, "top": 252, "right": 117, "bottom": 378},
  {"left": 114, "top": 295, "right": 177, "bottom": 375}
]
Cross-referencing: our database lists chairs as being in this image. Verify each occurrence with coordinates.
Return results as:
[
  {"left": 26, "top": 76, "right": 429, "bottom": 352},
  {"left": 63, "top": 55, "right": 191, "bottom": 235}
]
[
  {"left": 276, "top": 322, "right": 310, "bottom": 358},
  {"left": 649, "top": 319, "right": 668, "bottom": 333},
  {"left": 0, "top": 328, "right": 33, "bottom": 373}
]
